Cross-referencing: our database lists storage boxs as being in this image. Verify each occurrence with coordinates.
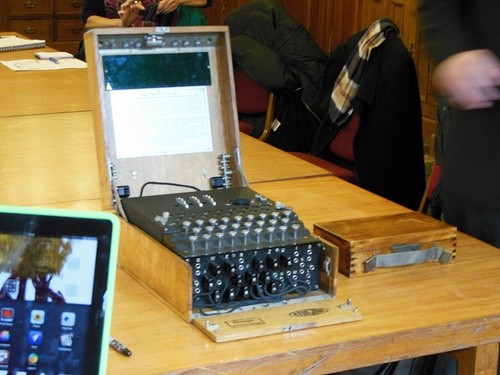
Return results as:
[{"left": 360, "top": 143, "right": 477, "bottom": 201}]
[
  {"left": 313, "top": 207, "right": 458, "bottom": 277},
  {"left": 82, "top": 24, "right": 341, "bottom": 322}
]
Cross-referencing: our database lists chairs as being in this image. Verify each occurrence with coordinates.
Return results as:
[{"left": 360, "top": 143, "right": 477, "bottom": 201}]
[
  {"left": 234, "top": 64, "right": 276, "bottom": 143},
  {"left": 287, "top": 109, "right": 361, "bottom": 182}
]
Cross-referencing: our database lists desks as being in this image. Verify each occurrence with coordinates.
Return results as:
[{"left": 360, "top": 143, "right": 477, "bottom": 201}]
[{"left": 0, "top": 27, "right": 500, "bottom": 375}]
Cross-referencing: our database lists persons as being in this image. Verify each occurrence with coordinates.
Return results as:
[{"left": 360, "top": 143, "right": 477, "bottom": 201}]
[
  {"left": 75, "top": 0, "right": 213, "bottom": 61},
  {"left": 419, "top": 1, "right": 500, "bottom": 250}
]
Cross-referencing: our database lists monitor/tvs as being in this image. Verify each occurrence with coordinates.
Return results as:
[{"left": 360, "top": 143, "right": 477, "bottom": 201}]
[{"left": 0, "top": 204, "right": 120, "bottom": 375}]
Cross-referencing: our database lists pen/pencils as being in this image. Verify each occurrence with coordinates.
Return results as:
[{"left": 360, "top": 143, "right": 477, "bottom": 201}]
[
  {"left": 108, "top": 336, "right": 132, "bottom": 357},
  {"left": 49, "top": 57, "right": 59, "bottom": 64},
  {"left": 0, "top": 35, "right": 19, "bottom": 39}
]
